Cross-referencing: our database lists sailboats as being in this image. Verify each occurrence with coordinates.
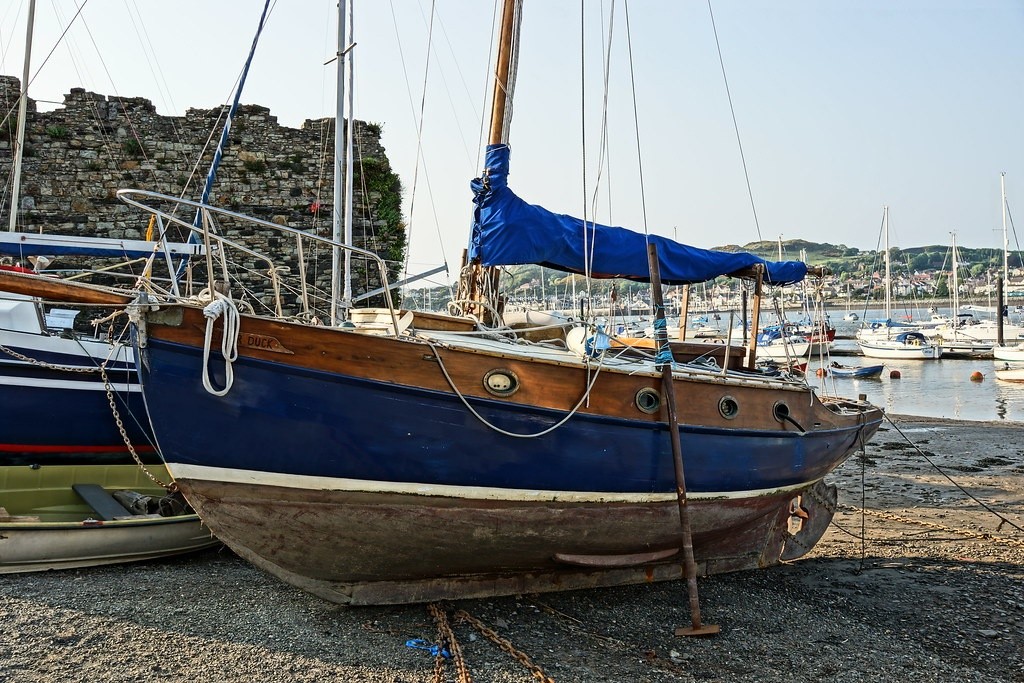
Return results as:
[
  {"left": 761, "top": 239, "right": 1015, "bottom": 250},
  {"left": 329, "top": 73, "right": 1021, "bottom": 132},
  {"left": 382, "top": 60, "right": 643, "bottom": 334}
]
[{"left": 0, "top": 2, "right": 1021, "bottom": 606}]
